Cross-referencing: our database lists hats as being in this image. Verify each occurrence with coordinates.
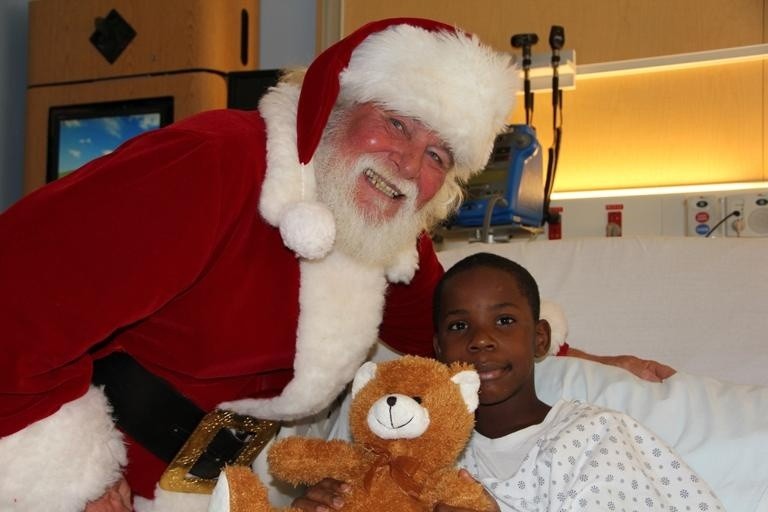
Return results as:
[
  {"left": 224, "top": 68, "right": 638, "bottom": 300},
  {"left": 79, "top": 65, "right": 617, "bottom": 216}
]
[{"left": 292, "top": 14, "right": 526, "bottom": 184}]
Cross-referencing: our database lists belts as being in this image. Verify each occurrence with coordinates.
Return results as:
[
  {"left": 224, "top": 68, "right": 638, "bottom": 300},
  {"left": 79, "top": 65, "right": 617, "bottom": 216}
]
[{"left": 83, "top": 346, "right": 285, "bottom": 499}]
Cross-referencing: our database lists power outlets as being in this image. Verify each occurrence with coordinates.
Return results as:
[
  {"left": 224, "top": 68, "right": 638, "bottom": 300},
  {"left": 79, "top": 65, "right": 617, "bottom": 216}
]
[{"left": 724, "top": 195, "right": 768, "bottom": 238}]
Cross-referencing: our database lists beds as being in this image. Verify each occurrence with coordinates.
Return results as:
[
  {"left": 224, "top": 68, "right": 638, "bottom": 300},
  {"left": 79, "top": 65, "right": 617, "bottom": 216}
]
[{"left": 363, "top": 234, "right": 768, "bottom": 512}]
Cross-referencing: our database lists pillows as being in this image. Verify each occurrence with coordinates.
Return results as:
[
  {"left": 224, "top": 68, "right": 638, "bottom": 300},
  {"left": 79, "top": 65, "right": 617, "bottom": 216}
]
[{"left": 532, "top": 355, "right": 768, "bottom": 512}]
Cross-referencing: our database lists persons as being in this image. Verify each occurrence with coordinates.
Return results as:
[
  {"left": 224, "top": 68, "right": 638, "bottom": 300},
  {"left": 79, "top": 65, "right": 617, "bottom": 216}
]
[
  {"left": 0, "top": 13, "right": 677, "bottom": 511},
  {"left": 288, "top": 250, "right": 724, "bottom": 512}
]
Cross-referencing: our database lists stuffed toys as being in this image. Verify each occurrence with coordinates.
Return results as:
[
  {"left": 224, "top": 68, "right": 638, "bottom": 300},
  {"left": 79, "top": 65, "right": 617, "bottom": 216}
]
[{"left": 201, "top": 352, "right": 502, "bottom": 512}]
